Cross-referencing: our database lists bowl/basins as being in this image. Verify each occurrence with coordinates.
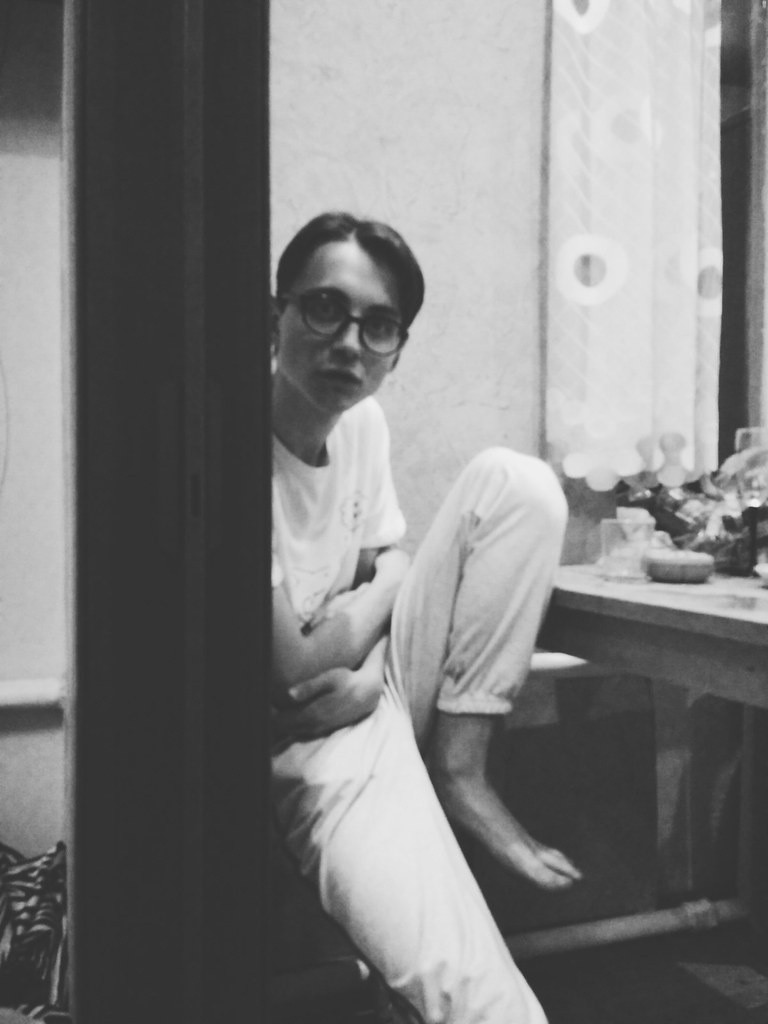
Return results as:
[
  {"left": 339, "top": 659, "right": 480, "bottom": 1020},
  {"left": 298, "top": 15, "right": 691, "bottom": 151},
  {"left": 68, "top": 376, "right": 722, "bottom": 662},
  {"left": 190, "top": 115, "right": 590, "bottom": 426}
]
[{"left": 639, "top": 551, "right": 714, "bottom": 583}]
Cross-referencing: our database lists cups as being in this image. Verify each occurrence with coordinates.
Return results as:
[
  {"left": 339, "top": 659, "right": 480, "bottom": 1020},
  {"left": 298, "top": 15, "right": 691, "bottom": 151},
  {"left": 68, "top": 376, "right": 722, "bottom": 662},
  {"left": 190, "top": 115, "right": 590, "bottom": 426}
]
[
  {"left": 735, "top": 428, "right": 768, "bottom": 508},
  {"left": 602, "top": 519, "right": 656, "bottom": 583}
]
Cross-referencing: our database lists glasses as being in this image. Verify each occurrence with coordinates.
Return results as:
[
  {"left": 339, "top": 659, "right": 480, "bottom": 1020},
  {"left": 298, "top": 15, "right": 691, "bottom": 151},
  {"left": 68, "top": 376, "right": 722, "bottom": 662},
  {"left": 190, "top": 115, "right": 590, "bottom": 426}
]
[{"left": 281, "top": 291, "right": 408, "bottom": 357}]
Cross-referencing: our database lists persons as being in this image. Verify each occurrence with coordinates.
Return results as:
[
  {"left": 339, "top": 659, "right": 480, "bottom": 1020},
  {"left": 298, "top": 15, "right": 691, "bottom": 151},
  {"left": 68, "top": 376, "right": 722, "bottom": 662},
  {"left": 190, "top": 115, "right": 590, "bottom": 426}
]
[{"left": 272, "top": 213, "right": 583, "bottom": 1024}]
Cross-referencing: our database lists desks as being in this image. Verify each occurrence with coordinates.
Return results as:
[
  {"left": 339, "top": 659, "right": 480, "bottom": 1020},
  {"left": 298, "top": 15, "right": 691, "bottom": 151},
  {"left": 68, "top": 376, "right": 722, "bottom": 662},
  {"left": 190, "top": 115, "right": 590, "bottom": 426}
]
[{"left": 534, "top": 564, "right": 768, "bottom": 1017}]
